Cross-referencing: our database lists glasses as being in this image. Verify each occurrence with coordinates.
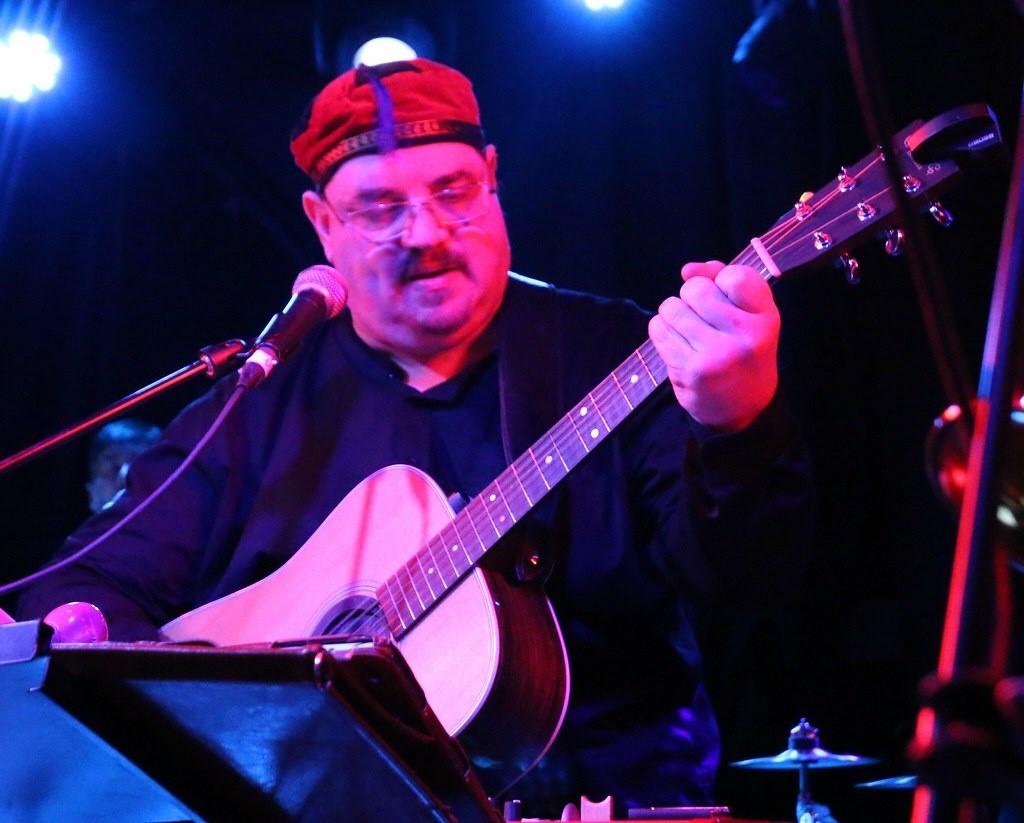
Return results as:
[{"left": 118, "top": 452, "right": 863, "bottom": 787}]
[{"left": 324, "top": 167, "right": 489, "bottom": 243}]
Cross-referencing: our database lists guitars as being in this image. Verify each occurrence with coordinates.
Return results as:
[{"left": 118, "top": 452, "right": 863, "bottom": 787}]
[{"left": 142, "top": 99, "right": 1008, "bottom": 802}]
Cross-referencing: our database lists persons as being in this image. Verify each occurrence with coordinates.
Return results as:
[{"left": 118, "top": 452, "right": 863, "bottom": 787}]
[{"left": 22, "top": 58, "right": 816, "bottom": 820}]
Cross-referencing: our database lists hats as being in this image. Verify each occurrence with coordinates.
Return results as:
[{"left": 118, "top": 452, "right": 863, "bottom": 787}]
[{"left": 289, "top": 58, "right": 485, "bottom": 185}]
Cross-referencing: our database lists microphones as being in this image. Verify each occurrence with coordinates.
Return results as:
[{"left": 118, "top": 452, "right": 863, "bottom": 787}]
[{"left": 237, "top": 264, "right": 349, "bottom": 394}]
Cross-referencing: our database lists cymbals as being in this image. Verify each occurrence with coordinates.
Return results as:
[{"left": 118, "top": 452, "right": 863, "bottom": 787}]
[
  {"left": 725, "top": 717, "right": 879, "bottom": 774},
  {"left": 852, "top": 772, "right": 919, "bottom": 791}
]
